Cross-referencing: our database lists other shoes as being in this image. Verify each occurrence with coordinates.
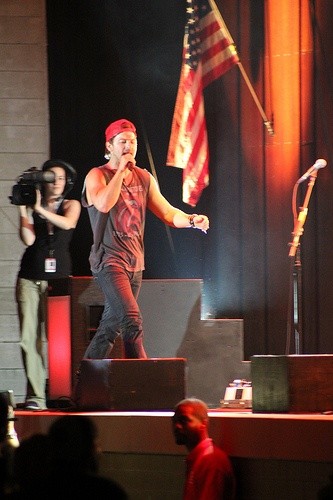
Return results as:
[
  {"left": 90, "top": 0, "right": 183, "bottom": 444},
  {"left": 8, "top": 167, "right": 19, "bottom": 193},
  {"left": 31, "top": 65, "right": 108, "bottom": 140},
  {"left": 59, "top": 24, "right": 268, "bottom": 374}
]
[{"left": 23, "top": 398, "right": 47, "bottom": 410}]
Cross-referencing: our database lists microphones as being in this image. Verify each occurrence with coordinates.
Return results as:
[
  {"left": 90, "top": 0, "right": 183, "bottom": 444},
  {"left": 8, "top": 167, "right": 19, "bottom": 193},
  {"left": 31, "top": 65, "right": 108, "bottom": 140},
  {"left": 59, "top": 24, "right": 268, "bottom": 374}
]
[{"left": 123, "top": 153, "right": 133, "bottom": 171}]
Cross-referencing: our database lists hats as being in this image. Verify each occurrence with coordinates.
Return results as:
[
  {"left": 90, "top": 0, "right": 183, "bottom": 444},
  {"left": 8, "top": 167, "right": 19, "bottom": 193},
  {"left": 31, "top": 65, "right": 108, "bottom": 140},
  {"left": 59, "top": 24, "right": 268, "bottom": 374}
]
[{"left": 105, "top": 119, "right": 137, "bottom": 143}]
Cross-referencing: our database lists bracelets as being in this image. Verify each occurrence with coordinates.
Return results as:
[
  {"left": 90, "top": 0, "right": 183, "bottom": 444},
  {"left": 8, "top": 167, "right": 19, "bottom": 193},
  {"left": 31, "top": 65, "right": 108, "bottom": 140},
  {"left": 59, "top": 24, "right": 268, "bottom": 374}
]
[{"left": 189, "top": 214, "right": 195, "bottom": 228}]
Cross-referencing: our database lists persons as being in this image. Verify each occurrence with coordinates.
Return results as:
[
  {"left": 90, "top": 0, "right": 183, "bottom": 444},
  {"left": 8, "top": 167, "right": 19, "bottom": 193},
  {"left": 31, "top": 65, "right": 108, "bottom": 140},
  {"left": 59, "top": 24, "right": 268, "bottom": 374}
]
[
  {"left": 1, "top": 393, "right": 128, "bottom": 500},
  {"left": 82, "top": 119, "right": 210, "bottom": 359},
  {"left": 172, "top": 399, "right": 236, "bottom": 500},
  {"left": 16, "top": 158, "right": 81, "bottom": 410}
]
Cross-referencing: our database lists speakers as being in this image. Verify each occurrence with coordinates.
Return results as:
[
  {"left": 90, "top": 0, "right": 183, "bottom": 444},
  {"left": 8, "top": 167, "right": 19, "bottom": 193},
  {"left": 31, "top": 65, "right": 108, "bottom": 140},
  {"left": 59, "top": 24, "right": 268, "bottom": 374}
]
[
  {"left": 78, "top": 358, "right": 187, "bottom": 411},
  {"left": 251, "top": 354, "right": 333, "bottom": 414},
  {"left": 296, "top": 158, "right": 327, "bottom": 184}
]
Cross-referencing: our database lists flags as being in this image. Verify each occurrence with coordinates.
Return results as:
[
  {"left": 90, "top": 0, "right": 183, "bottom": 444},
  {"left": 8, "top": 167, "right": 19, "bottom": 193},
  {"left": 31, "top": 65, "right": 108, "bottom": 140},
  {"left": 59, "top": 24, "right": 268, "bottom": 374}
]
[{"left": 165, "top": 0, "right": 240, "bottom": 208}]
